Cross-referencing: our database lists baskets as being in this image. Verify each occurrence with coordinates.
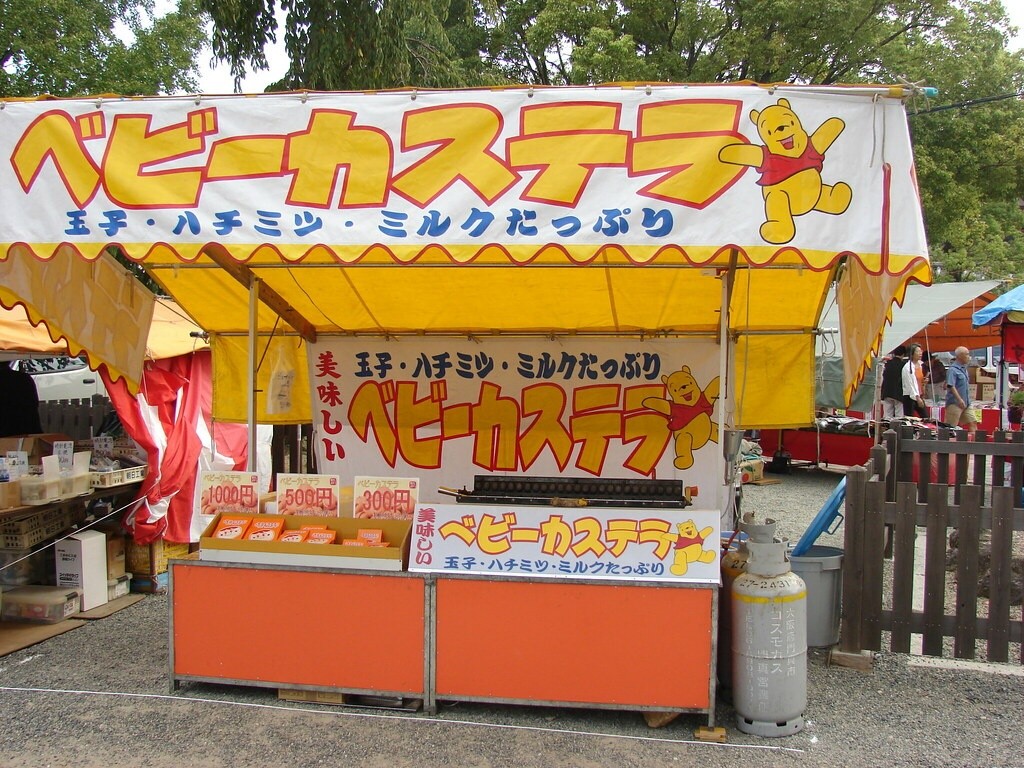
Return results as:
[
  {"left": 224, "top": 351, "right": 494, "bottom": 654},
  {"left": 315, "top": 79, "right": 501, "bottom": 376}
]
[{"left": 0, "top": 435, "right": 148, "bottom": 550}]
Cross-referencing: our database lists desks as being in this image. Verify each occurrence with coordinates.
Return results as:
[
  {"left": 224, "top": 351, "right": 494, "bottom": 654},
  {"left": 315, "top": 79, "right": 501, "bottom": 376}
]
[
  {"left": 741, "top": 429, "right": 781, "bottom": 473},
  {"left": 781, "top": 418, "right": 971, "bottom": 485},
  {"left": 167, "top": 553, "right": 429, "bottom": 716},
  {"left": 846, "top": 403, "right": 1022, "bottom": 463},
  {"left": 430, "top": 570, "right": 725, "bottom": 733}
]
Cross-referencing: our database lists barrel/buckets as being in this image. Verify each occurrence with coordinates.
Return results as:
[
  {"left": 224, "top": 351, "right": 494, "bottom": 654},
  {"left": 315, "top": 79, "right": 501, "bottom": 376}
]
[{"left": 784, "top": 545, "right": 843, "bottom": 647}]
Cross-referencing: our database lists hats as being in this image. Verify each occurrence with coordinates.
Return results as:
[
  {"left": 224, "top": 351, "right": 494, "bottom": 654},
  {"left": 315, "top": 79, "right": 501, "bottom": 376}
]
[{"left": 921, "top": 351, "right": 933, "bottom": 363}]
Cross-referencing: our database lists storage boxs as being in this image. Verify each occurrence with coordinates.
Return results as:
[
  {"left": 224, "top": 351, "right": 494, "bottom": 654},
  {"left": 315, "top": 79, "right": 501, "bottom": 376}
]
[
  {"left": 106, "top": 535, "right": 125, "bottom": 580},
  {"left": 20, "top": 471, "right": 91, "bottom": 506},
  {"left": 0, "top": 515, "right": 71, "bottom": 550},
  {"left": 740, "top": 461, "right": 764, "bottom": 483},
  {"left": 968, "top": 366, "right": 996, "bottom": 383},
  {"left": 107, "top": 573, "right": 133, "bottom": 601},
  {"left": 199, "top": 512, "right": 413, "bottom": 571},
  {"left": 969, "top": 384, "right": 977, "bottom": 400},
  {"left": 977, "top": 384, "right": 994, "bottom": 401},
  {"left": 0, "top": 503, "right": 68, "bottom": 535},
  {"left": 0, "top": 433, "right": 71, "bottom": 465},
  {"left": 927, "top": 382, "right": 947, "bottom": 400},
  {"left": 55, "top": 530, "right": 108, "bottom": 612},
  {"left": 89, "top": 464, "right": 148, "bottom": 488}
]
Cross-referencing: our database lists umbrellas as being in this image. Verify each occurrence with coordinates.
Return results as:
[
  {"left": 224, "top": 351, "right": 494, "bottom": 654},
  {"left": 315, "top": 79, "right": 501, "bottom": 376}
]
[{"left": 970, "top": 284, "right": 1024, "bottom": 329}]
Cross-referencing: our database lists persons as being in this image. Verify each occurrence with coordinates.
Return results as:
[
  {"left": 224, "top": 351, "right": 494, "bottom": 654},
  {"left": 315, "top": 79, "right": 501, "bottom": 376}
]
[
  {"left": 881, "top": 343, "right": 930, "bottom": 420},
  {"left": 945, "top": 346, "right": 978, "bottom": 432}
]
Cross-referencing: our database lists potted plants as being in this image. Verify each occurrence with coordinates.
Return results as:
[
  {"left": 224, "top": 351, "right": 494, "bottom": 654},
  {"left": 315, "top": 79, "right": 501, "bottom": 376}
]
[{"left": 1007, "top": 391, "right": 1023, "bottom": 424}]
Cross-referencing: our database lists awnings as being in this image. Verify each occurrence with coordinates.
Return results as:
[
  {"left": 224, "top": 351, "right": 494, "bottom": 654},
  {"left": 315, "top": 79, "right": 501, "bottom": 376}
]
[
  {"left": 814, "top": 279, "right": 1003, "bottom": 363},
  {"left": 0, "top": 80, "right": 933, "bottom": 430},
  {"left": 0, "top": 292, "right": 210, "bottom": 362}
]
